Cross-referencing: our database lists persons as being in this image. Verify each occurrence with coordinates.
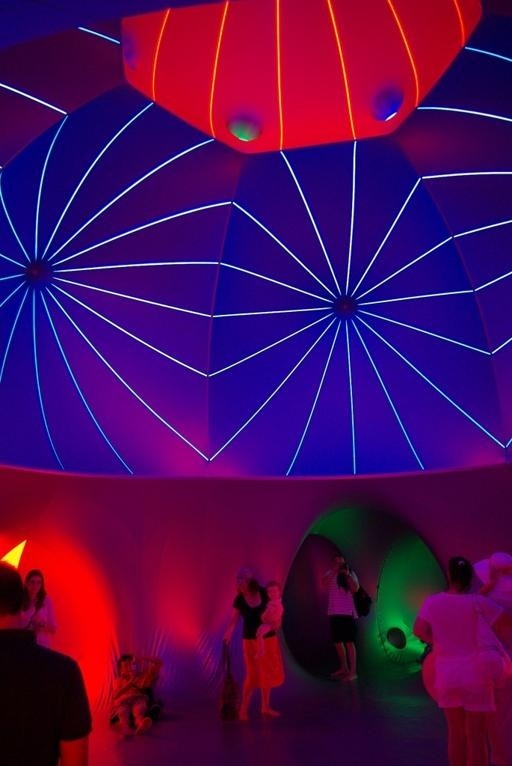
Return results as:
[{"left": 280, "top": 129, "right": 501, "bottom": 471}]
[
  {"left": 1, "top": 562, "right": 94, "bottom": 764},
  {"left": 223, "top": 568, "right": 283, "bottom": 722},
  {"left": 319, "top": 552, "right": 361, "bottom": 684},
  {"left": 112, "top": 654, "right": 152, "bottom": 738},
  {"left": 23, "top": 568, "right": 61, "bottom": 654},
  {"left": 250, "top": 581, "right": 285, "bottom": 662},
  {"left": 105, "top": 657, "right": 165, "bottom": 726},
  {"left": 411, "top": 555, "right": 510, "bottom": 764}
]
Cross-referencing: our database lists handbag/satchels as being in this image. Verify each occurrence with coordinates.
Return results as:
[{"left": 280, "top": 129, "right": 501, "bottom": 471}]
[{"left": 215, "top": 640, "right": 242, "bottom": 722}]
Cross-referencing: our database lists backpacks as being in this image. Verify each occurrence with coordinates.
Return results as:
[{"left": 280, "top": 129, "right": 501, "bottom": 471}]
[{"left": 351, "top": 584, "right": 371, "bottom": 618}]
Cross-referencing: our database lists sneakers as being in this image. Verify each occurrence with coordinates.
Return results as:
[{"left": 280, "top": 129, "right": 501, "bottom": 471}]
[
  {"left": 240, "top": 709, "right": 250, "bottom": 723},
  {"left": 332, "top": 665, "right": 356, "bottom": 681},
  {"left": 260, "top": 706, "right": 280, "bottom": 718}
]
